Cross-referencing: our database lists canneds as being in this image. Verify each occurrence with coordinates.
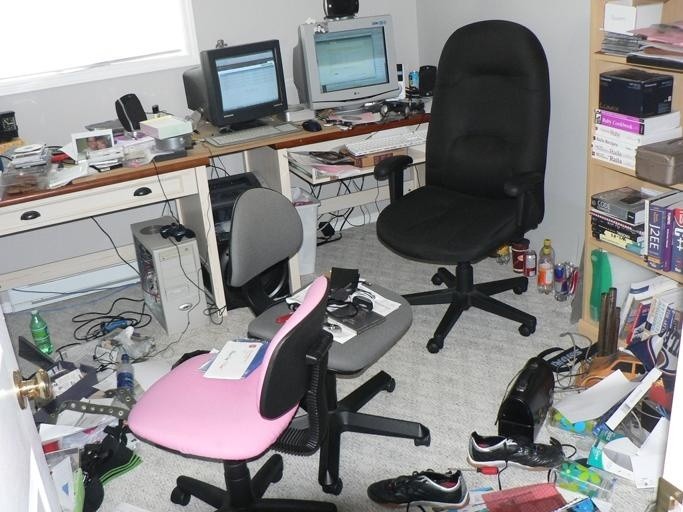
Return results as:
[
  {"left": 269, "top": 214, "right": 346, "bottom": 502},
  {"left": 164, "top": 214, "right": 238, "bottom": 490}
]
[
  {"left": 554, "top": 264, "right": 567, "bottom": 293},
  {"left": 523, "top": 251, "right": 537, "bottom": 277}
]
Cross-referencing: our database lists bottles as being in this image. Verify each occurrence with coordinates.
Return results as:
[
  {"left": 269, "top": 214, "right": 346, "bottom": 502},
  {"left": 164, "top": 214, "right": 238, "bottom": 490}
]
[
  {"left": 496, "top": 238, "right": 576, "bottom": 302},
  {"left": 549, "top": 456, "right": 613, "bottom": 500},
  {"left": 594, "top": 427, "right": 623, "bottom": 443},
  {"left": 409, "top": 69, "right": 418, "bottom": 93},
  {"left": 549, "top": 407, "right": 595, "bottom": 436},
  {"left": 587, "top": 445, "right": 605, "bottom": 470},
  {"left": 115, "top": 353, "right": 133, "bottom": 398},
  {"left": 101, "top": 320, "right": 126, "bottom": 335},
  {"left": 30, "top": 309, "right": 53, "bottom": 352}
]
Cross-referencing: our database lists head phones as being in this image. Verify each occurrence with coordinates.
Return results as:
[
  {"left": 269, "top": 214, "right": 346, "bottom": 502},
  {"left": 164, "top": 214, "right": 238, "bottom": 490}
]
[{"left": 379, "top": 100, "right": 425, "bottom": 116}]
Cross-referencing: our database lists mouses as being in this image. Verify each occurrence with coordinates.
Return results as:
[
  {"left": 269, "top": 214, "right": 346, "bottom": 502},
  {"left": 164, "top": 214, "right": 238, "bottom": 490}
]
[{"left": 302, "top": 119, "right": 322, "bottom": 132}]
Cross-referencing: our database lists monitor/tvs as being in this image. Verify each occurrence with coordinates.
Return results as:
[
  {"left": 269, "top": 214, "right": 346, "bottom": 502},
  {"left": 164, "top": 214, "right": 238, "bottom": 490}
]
[
  {"left": 292, "top": 14, "right": 399, "bottom": 116},
  {"left": 182, "top": 39, "right": 289, "bottom": 133}
]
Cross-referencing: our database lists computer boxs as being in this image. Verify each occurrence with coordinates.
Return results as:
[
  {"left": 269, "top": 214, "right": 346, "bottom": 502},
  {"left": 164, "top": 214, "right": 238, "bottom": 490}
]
[{"left": 130, "top": 215, "right": 211, "bottom": 336}]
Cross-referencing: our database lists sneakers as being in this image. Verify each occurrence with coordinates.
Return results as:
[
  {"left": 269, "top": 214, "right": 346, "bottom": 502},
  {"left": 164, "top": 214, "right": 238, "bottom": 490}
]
[
  {"left": 368, "top": 469, "right": 470, "bottom": 512},
  {"left": 467, "top": 431, "right": 565, "bottom": 491}
]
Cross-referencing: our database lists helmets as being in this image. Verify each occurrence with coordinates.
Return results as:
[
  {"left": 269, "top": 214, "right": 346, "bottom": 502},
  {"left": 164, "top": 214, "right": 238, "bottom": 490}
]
[{"left": 73, "top": 467, "right": 104, "bottom": 512}]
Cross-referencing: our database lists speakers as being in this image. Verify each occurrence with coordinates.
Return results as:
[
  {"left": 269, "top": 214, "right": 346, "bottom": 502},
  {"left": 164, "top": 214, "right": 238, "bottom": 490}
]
[
  {"left": 114, "top": 93, "right": 148, "bottom": 132},
  {"left": 207, "top": 171, "right": 291, "bottom": 312}
]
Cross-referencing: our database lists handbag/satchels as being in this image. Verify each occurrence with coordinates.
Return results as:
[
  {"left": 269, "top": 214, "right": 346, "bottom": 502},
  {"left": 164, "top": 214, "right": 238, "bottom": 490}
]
[{"left": 499, "top": 357, "right": 554, "bottom": 442}]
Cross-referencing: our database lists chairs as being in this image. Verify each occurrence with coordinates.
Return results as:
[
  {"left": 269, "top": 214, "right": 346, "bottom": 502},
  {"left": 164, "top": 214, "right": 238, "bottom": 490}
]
[
  {"left": 229, "top": 188, "right": 431, "bottom": 495},
  {"left": 374, "top": 20, "right": 550, "bottom": 352},
  {"left": 127, "top": 275, "right": 335, "bottom": 511}
]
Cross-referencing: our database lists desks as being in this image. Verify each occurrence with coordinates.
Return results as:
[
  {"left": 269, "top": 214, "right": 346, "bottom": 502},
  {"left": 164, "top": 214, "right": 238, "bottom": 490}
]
[
  {"left": 0, "top": 147, "right": 227, "bottom": 317},
  {"left": 200, "top": 95, "right": 432, "bottom": 297}
]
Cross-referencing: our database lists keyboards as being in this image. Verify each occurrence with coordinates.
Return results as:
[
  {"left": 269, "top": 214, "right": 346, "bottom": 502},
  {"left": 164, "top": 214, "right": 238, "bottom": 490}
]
[
  {"left": 343, "top": 130, "right": 428, "bottom": 155},
  {"left": 206, "top": 120, "right": 302, "bottom": 147}
]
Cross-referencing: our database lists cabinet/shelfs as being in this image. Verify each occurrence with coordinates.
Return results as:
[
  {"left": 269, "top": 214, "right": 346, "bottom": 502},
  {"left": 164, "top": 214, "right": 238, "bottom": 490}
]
[{"left": 578, "top": 0, "right": 683, "bottom": 355}]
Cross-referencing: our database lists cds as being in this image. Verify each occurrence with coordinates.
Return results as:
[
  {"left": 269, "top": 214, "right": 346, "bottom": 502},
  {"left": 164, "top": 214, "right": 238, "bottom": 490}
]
[
  {"left": 141, "top": 224, "right": 162, "bottom": 234},
  {"left": 14, "top": 143, "right": 43, "bottom": 153}
]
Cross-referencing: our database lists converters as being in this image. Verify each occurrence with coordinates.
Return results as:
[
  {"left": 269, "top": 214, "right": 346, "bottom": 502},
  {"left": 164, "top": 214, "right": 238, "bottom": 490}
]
[{"left": 318, "top": 221, "right": 335, "bottom": 237}]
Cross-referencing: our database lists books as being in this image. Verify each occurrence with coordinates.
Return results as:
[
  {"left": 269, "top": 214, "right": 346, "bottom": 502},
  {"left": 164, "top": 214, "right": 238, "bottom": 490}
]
[
  {"left": 326, "top": 303, "right": 386, "bottom": 337},
  {"left": 583, "top": 1, "right": 682, "bottom": 372}
]
